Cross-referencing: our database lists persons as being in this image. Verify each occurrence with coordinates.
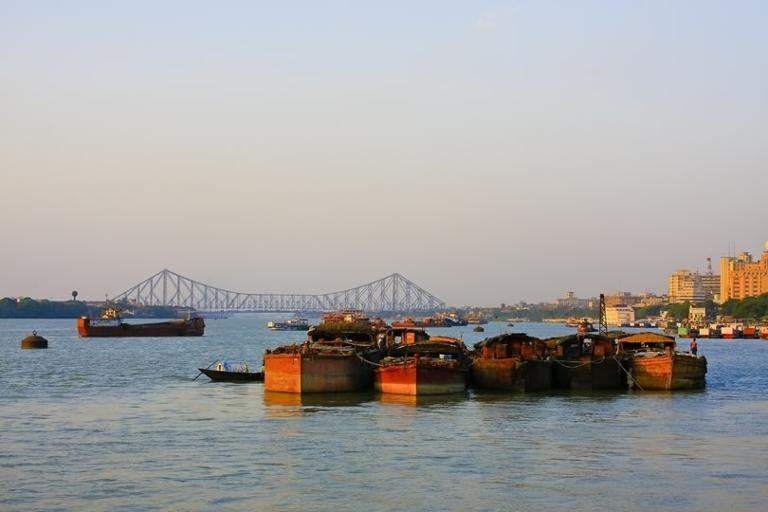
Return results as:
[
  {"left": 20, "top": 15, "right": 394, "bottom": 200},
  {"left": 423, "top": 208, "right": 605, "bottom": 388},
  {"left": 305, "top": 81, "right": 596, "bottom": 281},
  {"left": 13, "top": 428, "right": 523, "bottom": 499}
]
[{"left": 690, "top": 339, "right": 697, "bottom": 355}]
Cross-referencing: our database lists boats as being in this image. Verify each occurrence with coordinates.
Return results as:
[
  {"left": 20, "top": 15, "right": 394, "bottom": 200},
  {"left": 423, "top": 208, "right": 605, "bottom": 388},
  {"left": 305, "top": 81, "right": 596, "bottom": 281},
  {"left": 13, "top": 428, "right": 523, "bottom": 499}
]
[
  {"left": 77, "top": 318, "right": 204, "bottom": 337},
  {"left": 678, "top": 321, "right": 768, "bottom": 338},
  {"left": 21, "top": 330, "right": 47, "bottom": 349}
]
